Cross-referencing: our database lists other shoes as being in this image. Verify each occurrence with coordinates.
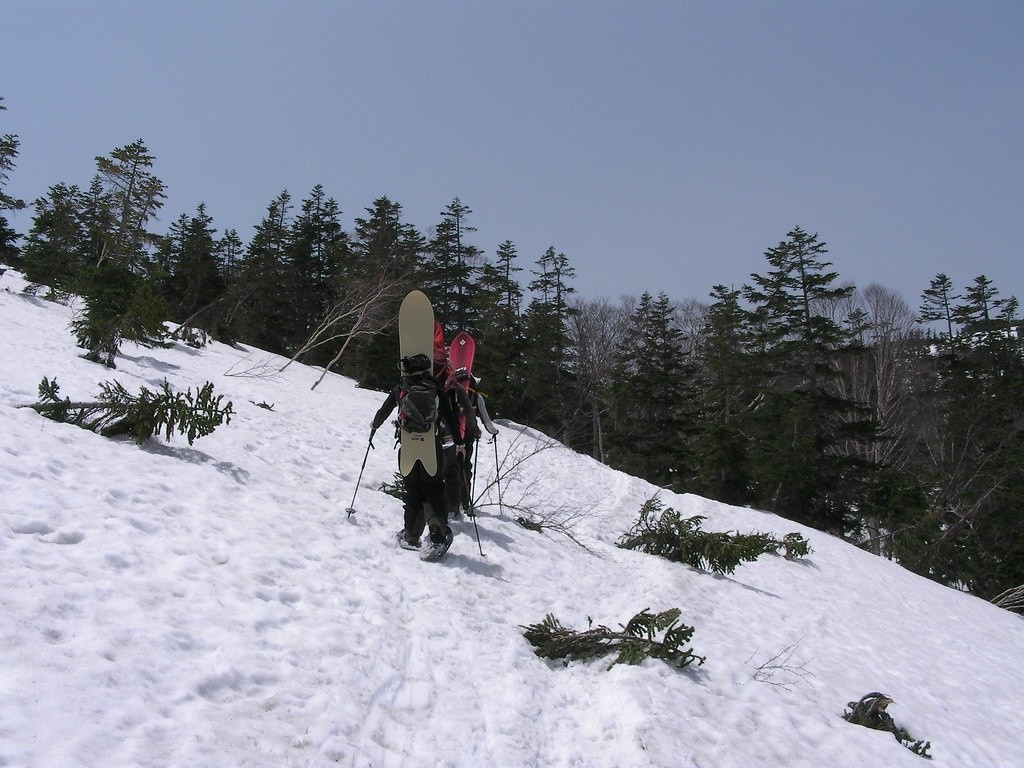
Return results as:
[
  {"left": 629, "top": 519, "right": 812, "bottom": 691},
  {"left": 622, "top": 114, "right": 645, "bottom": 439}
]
[
  {"left": 447, "top": 505, "right": 460, "bottom": 516},
  {"left": 398, "top": 529, "right": 422, "bottom": 550},
  {"left": 420, "top": 531, "right": 454, "bottom": 561},
  {"left": 463, "top": 497, "right": 469, "bottom": 511}
]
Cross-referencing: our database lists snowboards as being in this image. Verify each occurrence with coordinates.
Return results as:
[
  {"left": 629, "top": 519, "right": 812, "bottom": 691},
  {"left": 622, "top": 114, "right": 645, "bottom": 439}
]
[
  {"left": 397, "top": 289, "right": 442, "bottom": 480},
  {"left": 433, "top": 320, "right": 458, "bottom": 443},
  {"left": 448, "top": 331, "right": 476, "bottom": 442}
]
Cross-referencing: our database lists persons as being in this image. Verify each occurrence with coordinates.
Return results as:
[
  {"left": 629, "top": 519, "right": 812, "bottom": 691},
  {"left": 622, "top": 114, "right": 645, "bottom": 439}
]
[
  {"left": 400, "top": 357, "right": 499, "bottom": 525},
  {"left": 371, "top": 354, "right": 457, "bottom": 562}
]
